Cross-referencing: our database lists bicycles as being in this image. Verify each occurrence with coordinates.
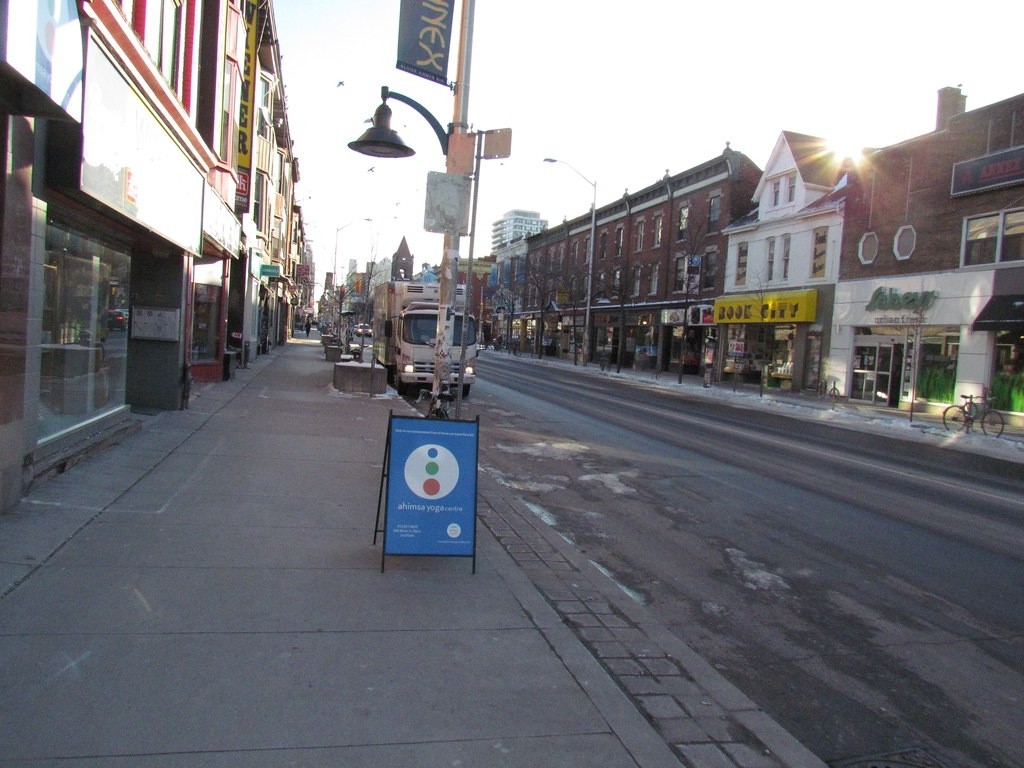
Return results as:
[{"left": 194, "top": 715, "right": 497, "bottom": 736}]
[
  {"left": 64, "top": 327, "right": 105, "bottom": 359},
  {"left": 943, "top": 395, "right": 1004, "bottom": 438}
]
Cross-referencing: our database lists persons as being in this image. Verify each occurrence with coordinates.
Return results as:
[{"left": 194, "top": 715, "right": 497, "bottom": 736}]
[
  {"left": 497, "top": 335, "right": 502, "bottom": 344},
  {"left": 305, "top": 320, "right": 311, "bottom": 337}
]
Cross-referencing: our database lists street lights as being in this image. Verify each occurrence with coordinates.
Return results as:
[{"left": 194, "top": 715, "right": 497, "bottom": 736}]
[
  {"left": 348, "top": 86, "right": 469, "bottom": 413},
  {"left": 332, "top": 218, "right": 373, "bottom": 332},
  {"left": 544, "top": 158, "right": 597, "bottom": 367}
]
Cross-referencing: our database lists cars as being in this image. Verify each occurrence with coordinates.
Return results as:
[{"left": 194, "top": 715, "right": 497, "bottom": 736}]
[
  {"left": 492, "top": 334, "right": 514, "bottom": 349},
  {"left": 357, "top": 324, "right": 372, "bottom": 337},
  {"left": 101, "top": 310, "right": 126, "bottom": 330}
]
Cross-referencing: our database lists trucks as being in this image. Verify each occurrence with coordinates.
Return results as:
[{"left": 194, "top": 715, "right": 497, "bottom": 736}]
[{"left": 373, "top": 282, "right": 491, "bottom": 396}]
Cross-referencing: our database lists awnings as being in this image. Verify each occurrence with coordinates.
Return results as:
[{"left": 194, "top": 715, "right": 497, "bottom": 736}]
[
  {"left": 270, "top": 274, "right": 289, "bottom": 284},
  {"left": 973, "top": 295, "right": 1024, "bottom": 330}
]
